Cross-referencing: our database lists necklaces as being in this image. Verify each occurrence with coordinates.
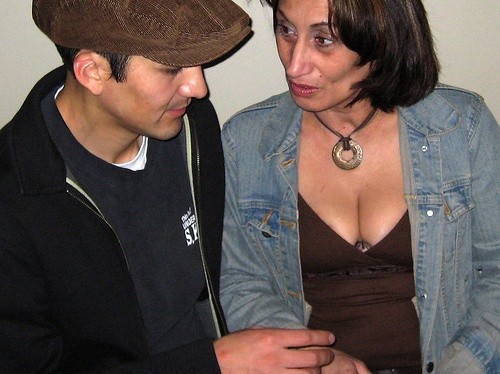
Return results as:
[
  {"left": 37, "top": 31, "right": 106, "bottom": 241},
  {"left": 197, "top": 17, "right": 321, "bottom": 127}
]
[{"left": 313, "top": 105, "right": 378, "bottom": 171}]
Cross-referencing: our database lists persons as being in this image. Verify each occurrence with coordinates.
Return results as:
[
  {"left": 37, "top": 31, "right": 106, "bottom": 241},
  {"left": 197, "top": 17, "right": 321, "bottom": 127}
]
[
  {"left": 218, "top": 0, "right": 500, "bottom": 374},
  {"left": 0, "top": 0, "right": 337, "bottom": 374}
]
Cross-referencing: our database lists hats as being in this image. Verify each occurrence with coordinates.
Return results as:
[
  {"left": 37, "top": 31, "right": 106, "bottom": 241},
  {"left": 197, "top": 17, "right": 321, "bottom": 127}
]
[{"left": 32, "top": 0, "right": 252, "bottom": 67}]
